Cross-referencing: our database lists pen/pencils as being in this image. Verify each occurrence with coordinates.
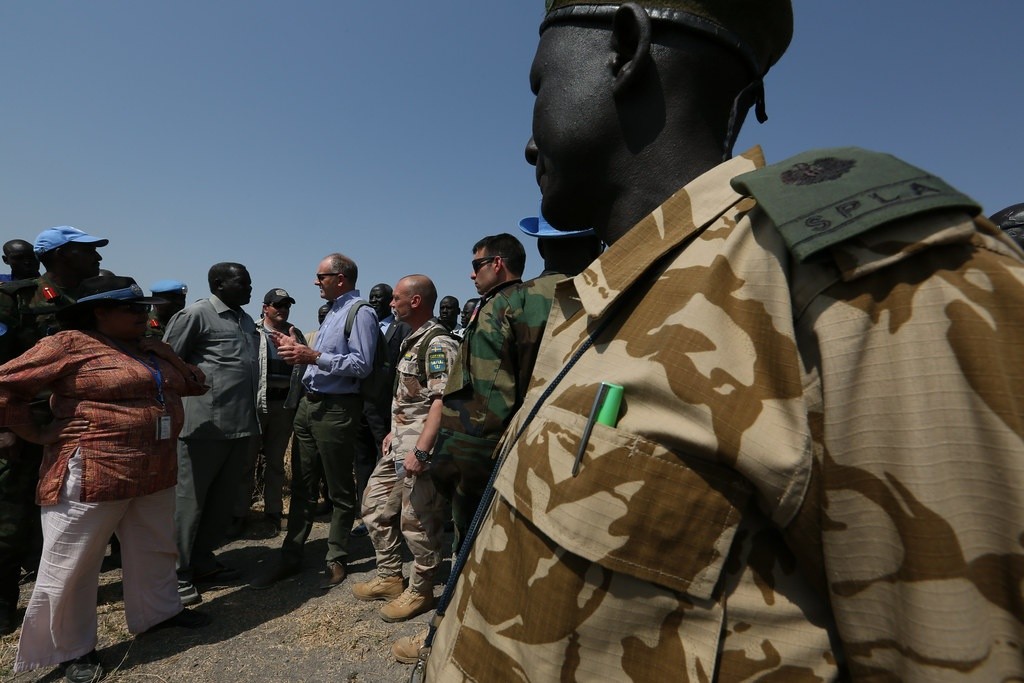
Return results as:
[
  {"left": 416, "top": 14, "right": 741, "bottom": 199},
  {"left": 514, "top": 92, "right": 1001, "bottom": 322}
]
[{"left": 573, "top": 381, "right": 625, "bottom": 475}]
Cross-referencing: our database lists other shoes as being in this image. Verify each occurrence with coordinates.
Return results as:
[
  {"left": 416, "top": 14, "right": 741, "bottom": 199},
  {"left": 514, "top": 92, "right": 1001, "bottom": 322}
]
[
  {"left": 249, "top": 566, "right": 296, "bottom": 588},
  {"left": 195, "top": 562, "right": 239, "bottom": 582},
  {"left": 320, "top": 561, "right": 345, "bottom": 589},
  {"left": 351, "top": 523, "right": 369, "bottom": 538},
  {"left": 264, "top": 513, "right": 280, "bottom": 530},
  {"left": 225, "top": 516, "right": 245, "bottom": 538},
  {"left": 0, "top": 602, "right": 18, "bottom": 633},
  {"left": 176, "top": 574, "right": 201, "bottom": 605}
]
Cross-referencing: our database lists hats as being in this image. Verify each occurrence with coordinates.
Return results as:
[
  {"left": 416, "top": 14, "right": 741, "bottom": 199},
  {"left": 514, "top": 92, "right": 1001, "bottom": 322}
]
[
  {"left": 34, "top": 226, "right": 108, "bottom": 258},
  {"left": 264, "top": 288, "right": 296, "bottom": 304},
  {"left": 57, "top": 276, "right": 171, "bottom": 326},
  {"left": 150, "top": 279, "right": 187, "bottom": 299},
  {"left": 518, "top": 196, "right": 593, "bottom": 236},
  {"left": 539, "top": 0, "right": 794, "bottom": 123}
]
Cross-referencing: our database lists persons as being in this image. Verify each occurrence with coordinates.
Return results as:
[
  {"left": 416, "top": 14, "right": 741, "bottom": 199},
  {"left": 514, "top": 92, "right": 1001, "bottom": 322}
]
[
  {"left": 291, "top": 305, "right": 333, "bottom": 516},
  {"left": 390, "top": 232, "right": 552, "bottom": 666},
  {"left": 162, "top": 262, "right": 262, "bottom": 606},
  {"left": 439, "top": 296, "right": 466, "bottom": 339},
  {"left": 351, "top": 272, "right": 460, "bottom": 622},
  {"left": 146, "top": 280, "right": 188, "bottom": 341},
  {"left": 461, "top": 298, "right": 482, "bottom": 327},
  {"left": 423, "top": 1, "right": 1024, "bottom": 682},
  {"left": 349, "top": 283, "right": 412, "bottom": 536},
  {"left": 0, "top": 239, "right": 44, "bottom": 365},
  {"left": 224, "top": 288, "right": 309, "bottom": 538},
  {"left": 988, "top": 202, "right": 1024, "bottom": 249},
  {"left": 0, "top": 276, "right": 212, "bottom": 682},
  {"left": 248, "top": 253, "right": 380, "bottom": 589},
  {"left": 0, "top": 224, "right": 110, "bottom": 634}
]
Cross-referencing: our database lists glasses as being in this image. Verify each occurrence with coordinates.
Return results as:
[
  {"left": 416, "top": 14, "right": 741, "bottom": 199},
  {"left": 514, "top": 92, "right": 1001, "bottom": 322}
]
[
  {"left": 317, "top": 273, "right": 347, "bottom": 281},
  {"left": 269, "top": 302, "right": 291, "bottom": 309},
  {"left": 104, "top": 304, "right": 151, "bottom": 314},
  {"left": 472, "top": 256, "right": 509, "bottom": 273}
]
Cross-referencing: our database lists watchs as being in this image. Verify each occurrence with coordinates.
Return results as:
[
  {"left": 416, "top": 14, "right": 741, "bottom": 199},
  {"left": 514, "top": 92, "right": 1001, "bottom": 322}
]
[
  {"left": 413, "top": 447, "right": 430, "bottom": 462},
  {"left": 315, "top": 353, "right": 322, "bottom": 366}
]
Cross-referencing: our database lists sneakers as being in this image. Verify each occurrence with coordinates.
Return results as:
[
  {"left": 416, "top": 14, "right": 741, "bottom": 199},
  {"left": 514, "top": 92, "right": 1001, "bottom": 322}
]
[
  {"left": 391, "top": 629, "right": 430, "bottom": 665},
  {"left": 379, "top": 589, "right": 434, "bottom": 623},
  {"left": 59, "top": 649, "right": 106, "bottom": 683},
  {"left": 145, "top": 608, "right": 210, "bottom": 633},
  {"left": 351, "top": 575, "right": 403, "bottom": 600}
]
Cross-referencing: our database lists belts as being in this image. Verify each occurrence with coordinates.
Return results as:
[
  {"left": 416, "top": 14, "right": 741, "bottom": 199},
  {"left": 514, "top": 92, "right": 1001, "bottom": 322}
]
[{"left": 306, "top": 389, "right": 359, "bottom": 401}]
